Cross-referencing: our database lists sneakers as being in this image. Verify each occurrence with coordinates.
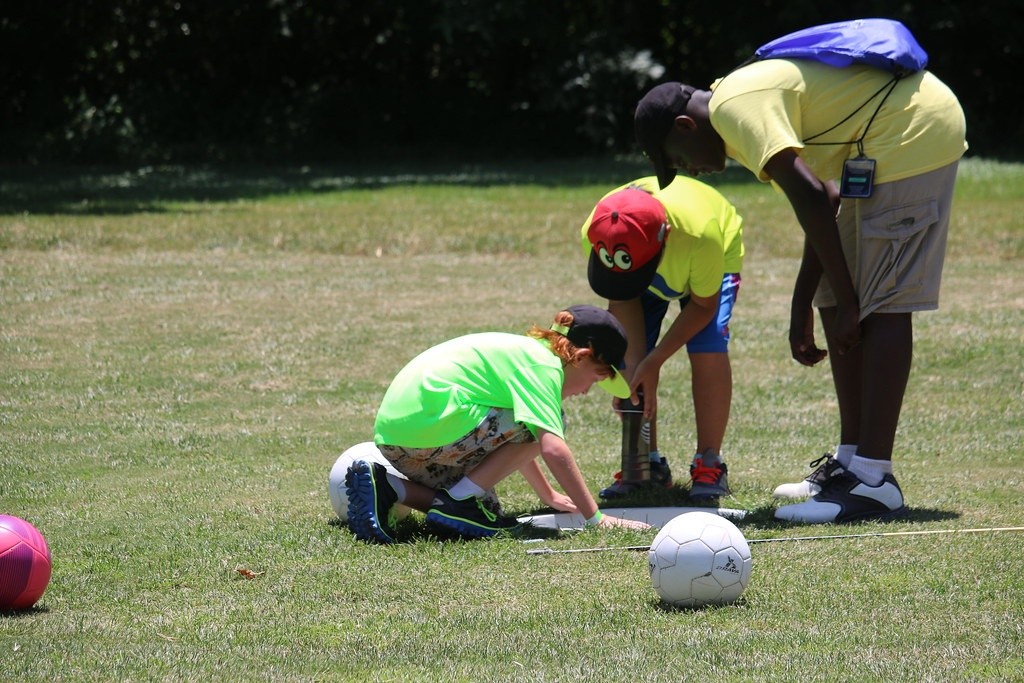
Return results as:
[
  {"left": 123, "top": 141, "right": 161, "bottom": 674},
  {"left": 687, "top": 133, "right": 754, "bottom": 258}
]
[
  {"left": 774, "top": 473, "right": 906, "bottom": 522},
  {"left": 426, "top": 488, "right": 522, "bottom": 539},
  {"left": 773, "top": 453, "right": 846, "bottom": 498},
  {"left": 687, "top": 453, "right": 728, "bottom": 499},
  {"left": 599, "top": 457, "right": 671, "bottom": 501},
  {"left": 345, "top": 460, "right": 400, "bottom": 544}
]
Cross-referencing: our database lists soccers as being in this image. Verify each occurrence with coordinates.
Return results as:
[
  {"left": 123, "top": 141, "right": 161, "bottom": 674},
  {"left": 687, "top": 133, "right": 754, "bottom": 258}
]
[
  {"left": 647, "top": 511, "right": 752, "bottom": 609},
  {"left": 0, "top": 513, "right": 52, "bottom": 614},
  {"left": 329, "top": 441, "right": 411, "bottom": 524}
]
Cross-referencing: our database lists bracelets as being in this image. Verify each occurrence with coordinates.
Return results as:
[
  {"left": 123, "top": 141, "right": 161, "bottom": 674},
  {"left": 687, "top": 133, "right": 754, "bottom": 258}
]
[{"left": 585, "top": 510, "right": 602, "bottom": 525}]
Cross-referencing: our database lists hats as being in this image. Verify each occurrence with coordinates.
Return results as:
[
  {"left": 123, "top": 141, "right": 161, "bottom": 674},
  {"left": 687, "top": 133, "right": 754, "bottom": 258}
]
[
  {"left": 550, "top": 304, "right": 632, "bottom": 399},
  {"left": 588, "top": 188, "right": 668, "bottom": 301},
  {"left": 633, "top": 82, "right": 695, "bottom": 191}
]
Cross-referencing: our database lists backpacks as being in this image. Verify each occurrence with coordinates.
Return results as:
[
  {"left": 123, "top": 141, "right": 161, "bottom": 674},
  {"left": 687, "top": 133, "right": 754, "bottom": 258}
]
[{"left": 734, "top": 17, "right": 928, "bottom": 146}]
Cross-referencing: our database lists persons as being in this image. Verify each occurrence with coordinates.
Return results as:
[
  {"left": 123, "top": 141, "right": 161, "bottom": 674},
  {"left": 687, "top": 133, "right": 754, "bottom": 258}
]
[
  {"left": 582, "top": 176, "right": 745, "bottom": 500},
  {"left": 345, "top": 304, "right": 653, "bottom": 544},
  {"left": 635, "top": 58, "right": 968, "bottom": 522}
]
